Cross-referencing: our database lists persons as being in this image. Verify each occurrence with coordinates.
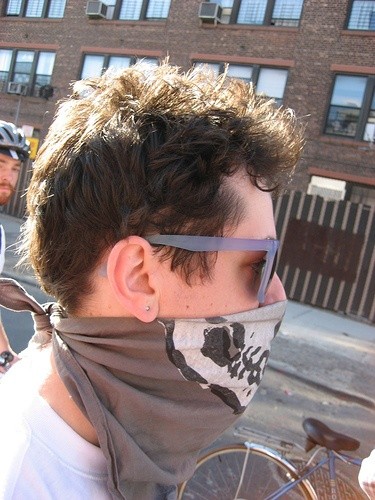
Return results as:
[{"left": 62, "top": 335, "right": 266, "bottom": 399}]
[
  {"left": 0, "top": 56, "right": 311, "bottom": 500},
  {"left": 0, "top": 119, "right": 34, "bottom": 379}
]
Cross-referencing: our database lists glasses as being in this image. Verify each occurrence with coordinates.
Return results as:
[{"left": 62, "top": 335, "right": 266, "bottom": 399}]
[{"left": 99, "top": 234, "right": 282, "bottom": 302}]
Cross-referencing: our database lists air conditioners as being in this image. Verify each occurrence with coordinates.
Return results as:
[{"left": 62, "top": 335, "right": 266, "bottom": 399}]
[
  {"left": 7, "top": 82, "right": 27, "bottom": 95},
  {"left": 86, "top": 1, "right": 108, "bottom": 19},
  {"left": 197, "top": 2, "right": 223, "bottom": 25}
]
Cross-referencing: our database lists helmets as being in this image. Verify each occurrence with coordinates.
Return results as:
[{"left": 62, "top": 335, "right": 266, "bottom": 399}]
[{"left": 0, "top": 118, "right": 31, "bottom": 159}]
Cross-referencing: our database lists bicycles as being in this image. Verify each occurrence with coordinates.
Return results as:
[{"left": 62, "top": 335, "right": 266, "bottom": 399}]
[{"left": 174, "top": 417, "right": 364, "bottom": 499}]
